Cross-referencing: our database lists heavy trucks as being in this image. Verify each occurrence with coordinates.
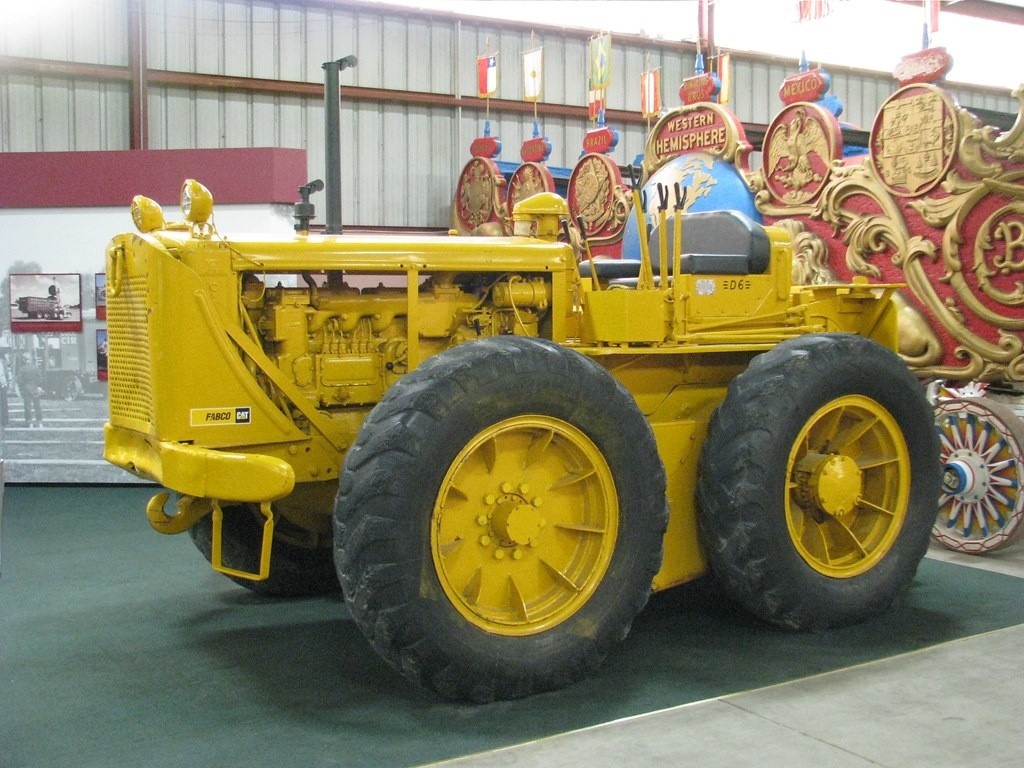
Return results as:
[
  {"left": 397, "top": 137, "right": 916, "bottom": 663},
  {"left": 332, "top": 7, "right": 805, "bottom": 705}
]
[
  {"left": 17, "top": 276, "right": 65, "bottom": 320},
  {"left": 36, "top": 308, "right": 110, "bottom": 404}
]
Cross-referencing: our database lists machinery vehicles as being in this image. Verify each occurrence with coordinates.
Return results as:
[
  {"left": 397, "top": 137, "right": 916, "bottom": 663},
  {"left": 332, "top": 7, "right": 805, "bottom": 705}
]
[{"left": 104, "top": 2, "right": 1021, "bottom": 704}]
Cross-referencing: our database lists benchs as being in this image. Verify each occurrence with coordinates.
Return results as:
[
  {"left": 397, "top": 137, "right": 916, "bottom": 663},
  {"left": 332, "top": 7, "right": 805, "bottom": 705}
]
[{"left": 577, "top": 207, "right": 772, "bottom": 289}]
